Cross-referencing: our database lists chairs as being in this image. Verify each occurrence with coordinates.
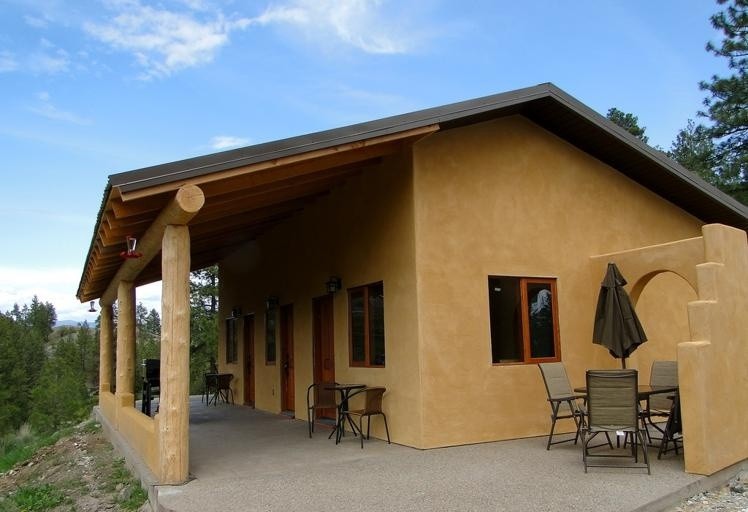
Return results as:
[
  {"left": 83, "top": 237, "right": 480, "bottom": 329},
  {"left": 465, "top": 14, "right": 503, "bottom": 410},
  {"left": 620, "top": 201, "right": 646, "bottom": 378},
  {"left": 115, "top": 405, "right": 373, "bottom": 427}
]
[
  {"left": 304, "top": 380, "right": 393, "bottom": 450},
  {"left": 139, "top": 358, "right": 160, "bottom": 416},
  {"left": 538, "top": 360, "right": 684, "bottom": 475},
  {"left": 202, "top": 372, "right": 234, "bottom": 408}
]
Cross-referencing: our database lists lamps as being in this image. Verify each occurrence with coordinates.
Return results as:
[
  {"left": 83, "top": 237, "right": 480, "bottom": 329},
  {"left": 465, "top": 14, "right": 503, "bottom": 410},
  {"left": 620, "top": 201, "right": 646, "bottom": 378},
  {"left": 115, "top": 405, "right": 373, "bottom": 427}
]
[
  {"left": 265, "top": 295, "right": 279, "bottom": 310},
  {"left": 231, "top": 305, "right": 243, "bottom": 319},
  {"left": 88, "top": 301, "right": 98, "bottom": 312},
  {"left": 119, "top": 236, "right": 143, "bottom": 258},
  {"left": 324, "top": 275, "right": 341, "bottom": 293}
]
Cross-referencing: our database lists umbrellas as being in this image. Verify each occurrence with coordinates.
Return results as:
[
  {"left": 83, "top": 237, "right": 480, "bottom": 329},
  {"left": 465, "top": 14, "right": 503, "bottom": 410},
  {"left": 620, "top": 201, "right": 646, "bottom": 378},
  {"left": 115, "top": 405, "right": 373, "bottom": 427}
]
[{"left": 591, "top": 261, "right": 650, "bottom": 368}]
